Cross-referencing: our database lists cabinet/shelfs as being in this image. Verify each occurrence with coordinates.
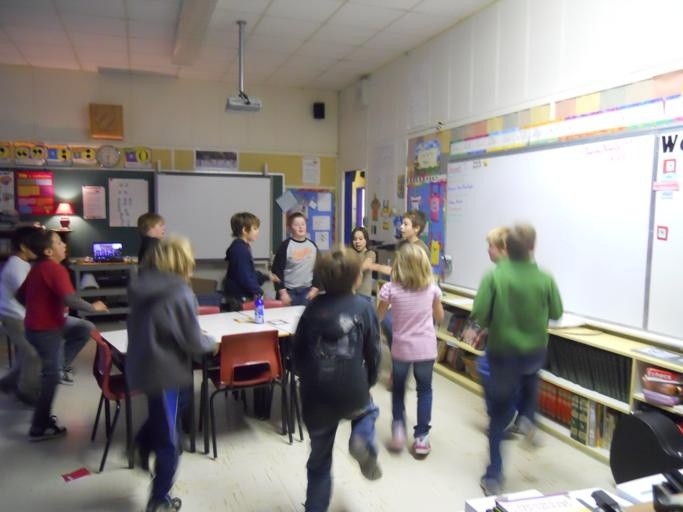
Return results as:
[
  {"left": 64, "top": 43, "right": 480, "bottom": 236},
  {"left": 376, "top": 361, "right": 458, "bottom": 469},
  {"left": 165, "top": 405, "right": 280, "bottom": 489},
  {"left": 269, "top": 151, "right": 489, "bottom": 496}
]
[
  {"left": 375, "top": 272, "right": 487, "bottom": 467},
  {"left": 533, "top": 302, "right": 683, "bottom": 469}
]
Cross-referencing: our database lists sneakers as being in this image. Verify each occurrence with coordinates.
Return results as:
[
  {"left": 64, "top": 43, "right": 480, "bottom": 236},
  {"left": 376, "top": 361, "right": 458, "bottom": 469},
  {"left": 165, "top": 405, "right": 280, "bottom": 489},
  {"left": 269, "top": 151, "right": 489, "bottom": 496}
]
[
  {"left": 29, "top": 415, "right": 66, "bottom": 441},
  {"left": 38, "top": 364, "right": 75, "bottom": 384},
  {"left": 412, "top": 435, "right": 432, "bottom": 456},
  {"left": 146, "top": 496, "right": 182, "bottom": 512}
]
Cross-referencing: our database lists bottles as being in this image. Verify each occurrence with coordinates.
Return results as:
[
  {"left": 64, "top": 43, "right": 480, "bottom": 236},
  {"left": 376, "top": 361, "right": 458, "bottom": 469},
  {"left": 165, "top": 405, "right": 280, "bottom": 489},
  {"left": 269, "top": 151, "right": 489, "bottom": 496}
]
[{"left": 254, "top": 291, "right": 264, "bottom": 324}]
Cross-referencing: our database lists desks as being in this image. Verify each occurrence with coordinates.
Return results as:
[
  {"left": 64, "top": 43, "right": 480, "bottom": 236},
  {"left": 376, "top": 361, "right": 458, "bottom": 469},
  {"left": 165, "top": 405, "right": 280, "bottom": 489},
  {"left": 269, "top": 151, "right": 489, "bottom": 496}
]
[
  {"left": 458, "top": 467, "right": 683, "bottom": 512},
  {"left": 70, "top": 258, "right": 132, "bottom": 320}
]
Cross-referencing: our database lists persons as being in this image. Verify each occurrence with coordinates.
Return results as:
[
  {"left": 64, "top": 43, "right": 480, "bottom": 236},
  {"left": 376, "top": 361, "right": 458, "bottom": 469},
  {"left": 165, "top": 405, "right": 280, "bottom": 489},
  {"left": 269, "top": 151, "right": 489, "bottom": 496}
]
[
  {"left": 126, "top": 240, "right": 219, "bottom": 512},
  {"left": 272, "top": 211, "right": 323, "bottom": 373},
  {"left": 471, "top": 226, "right": 524, "bottom": 433},
  {"left": 380, "top": 211, "right": 432, "bottom": 390},
  {"left": 222, "top": 212, "right": 281, "bottom": 311},
  {"left": 351, "top": 227, "right": 377, "bottom": 298},
  {"left": 138, "top": 213, "right": 167, "bottom": 270},
  {"left": 17, "top": 229, "right": 109, "bottom": 443},
  {"left": 291, "top": 246, "right": 381, "bottom": 512},
  {"left": 468, "top": 224, "right": 564, "bottom": 497},
  {"left": 377, "top": 241, "right": 444, "bottom": 456},
  {"left": 0, "top": 225, "right": 42, "bottom": 405}
]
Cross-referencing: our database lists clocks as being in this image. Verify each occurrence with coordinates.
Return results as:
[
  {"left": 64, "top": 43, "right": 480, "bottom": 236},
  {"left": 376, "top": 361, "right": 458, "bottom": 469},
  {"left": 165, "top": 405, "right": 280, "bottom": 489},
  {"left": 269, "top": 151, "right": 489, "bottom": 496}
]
[{"left": 96, "top": 145, "right": 120, "bottom": 167}]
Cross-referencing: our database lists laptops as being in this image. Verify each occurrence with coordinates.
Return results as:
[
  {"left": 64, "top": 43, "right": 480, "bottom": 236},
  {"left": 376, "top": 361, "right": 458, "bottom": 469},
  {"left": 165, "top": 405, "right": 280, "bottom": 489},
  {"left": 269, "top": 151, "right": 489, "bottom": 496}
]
[{"left": 92, "top": 241, "right": 125, "bottom": 263}]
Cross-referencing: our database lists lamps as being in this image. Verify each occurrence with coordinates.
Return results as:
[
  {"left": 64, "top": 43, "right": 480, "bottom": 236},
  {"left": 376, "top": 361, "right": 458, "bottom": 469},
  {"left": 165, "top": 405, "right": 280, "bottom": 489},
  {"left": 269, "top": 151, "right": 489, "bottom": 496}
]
[{"left": 55, "top": 202, "right": 74, "bottom": 229}]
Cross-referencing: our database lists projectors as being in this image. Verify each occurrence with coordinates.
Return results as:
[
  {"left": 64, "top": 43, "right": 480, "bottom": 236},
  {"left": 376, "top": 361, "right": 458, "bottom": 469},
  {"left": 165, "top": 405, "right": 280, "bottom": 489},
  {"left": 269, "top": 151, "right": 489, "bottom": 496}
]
[{"left": 225, "top": 94, "right": 262, "bottom": 112}]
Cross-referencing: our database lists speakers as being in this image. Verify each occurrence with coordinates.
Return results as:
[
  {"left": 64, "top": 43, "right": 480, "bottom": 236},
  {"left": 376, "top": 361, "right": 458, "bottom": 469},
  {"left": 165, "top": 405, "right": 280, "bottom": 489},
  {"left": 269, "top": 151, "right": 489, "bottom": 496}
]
[{"left": 313, "top": 102, "right": 326, "bottom": 119}]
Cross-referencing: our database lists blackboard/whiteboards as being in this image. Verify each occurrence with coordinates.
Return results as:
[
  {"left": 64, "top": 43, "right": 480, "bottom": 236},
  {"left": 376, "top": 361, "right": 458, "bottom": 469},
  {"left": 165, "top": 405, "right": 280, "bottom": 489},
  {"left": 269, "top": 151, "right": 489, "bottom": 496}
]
[
  {"left": 157, "top": 174, "right": 272, "bottom": 259},
  {"left": 442, "top": 126, "right": 682, "bottom": 342},
  {"left": 0, "top": 166, "right": 284, "bottom": 268}
]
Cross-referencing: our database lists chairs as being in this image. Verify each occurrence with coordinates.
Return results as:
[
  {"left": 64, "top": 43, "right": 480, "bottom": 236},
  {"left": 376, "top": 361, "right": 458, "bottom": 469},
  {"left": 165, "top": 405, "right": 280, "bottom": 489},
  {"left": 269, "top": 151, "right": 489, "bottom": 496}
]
[
  {"left": 91, "top": 293, "right": 374, "bottom": 472},
  {"left": 607, "top": 411, "right": 683, "bottom": 481}
]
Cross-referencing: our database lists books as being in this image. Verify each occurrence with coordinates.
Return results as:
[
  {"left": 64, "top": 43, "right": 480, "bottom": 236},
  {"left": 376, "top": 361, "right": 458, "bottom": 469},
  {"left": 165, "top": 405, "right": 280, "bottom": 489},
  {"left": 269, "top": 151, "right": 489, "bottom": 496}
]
[
  {"left": 539, "top": 338, "right": 634, "bottom": 450},
  {"left": 434, "top": 306, "right": 488, "bottom": 349},
  {"left": 466, "top": 472, "right": 666, "bottom": 512}
]
